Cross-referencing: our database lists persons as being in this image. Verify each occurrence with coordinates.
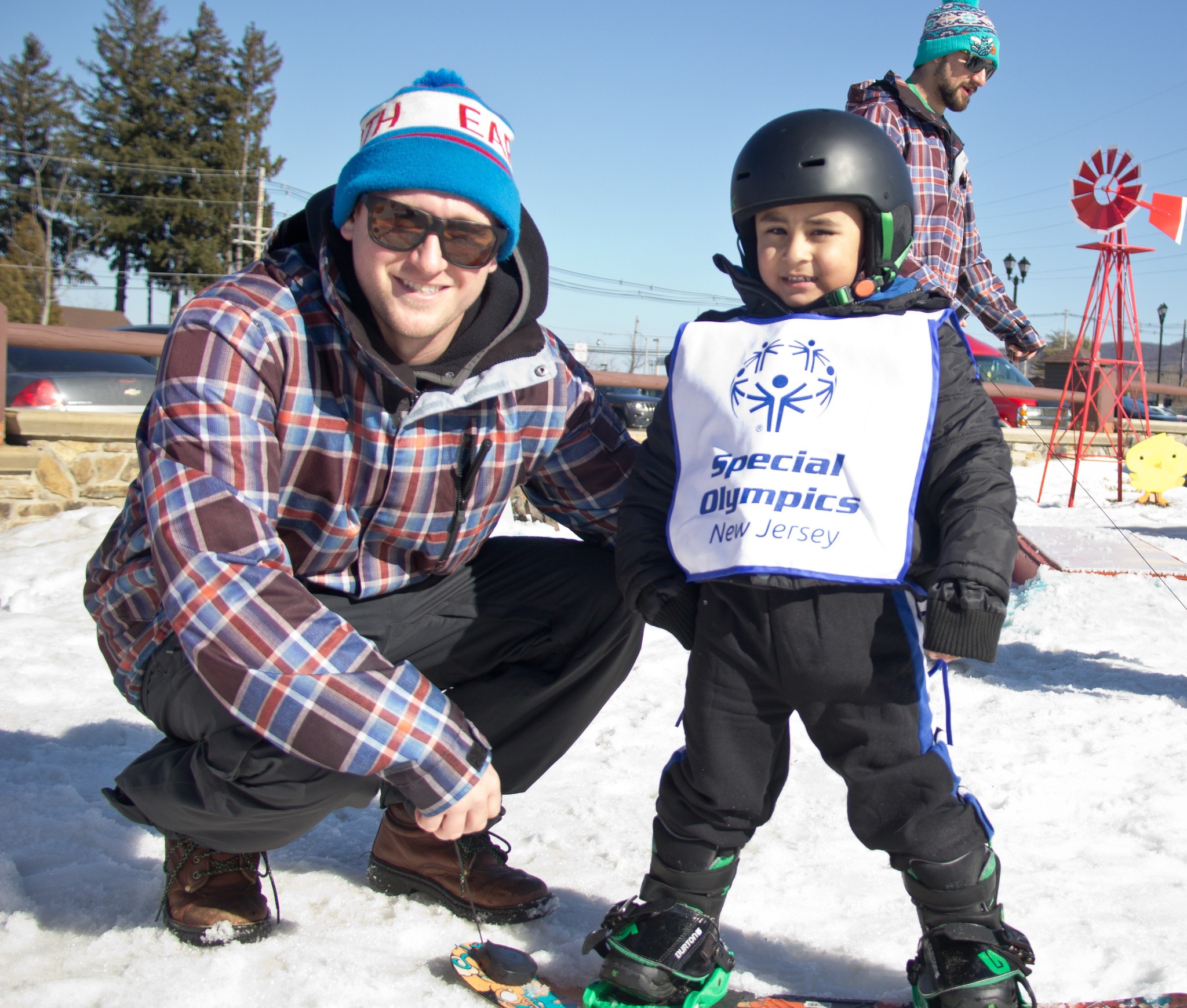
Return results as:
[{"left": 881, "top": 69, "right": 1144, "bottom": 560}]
[
  {"left": 582, "top": 108, "right": 1038, "bottom": 1008},
  {"left": 845, "top": 0, "right": 1047, "bottom": 361},
  {"left": 82, "top": 68, "right": 644, "bottom": 947}
]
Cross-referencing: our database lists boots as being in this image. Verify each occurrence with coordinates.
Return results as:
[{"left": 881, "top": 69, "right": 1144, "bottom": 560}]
[
  {"left": 901, "top": 845, "right": 1037, "bottom": 1008},
  {"left": 599, "top": 814, "right": 742, "bottom": 1003}
]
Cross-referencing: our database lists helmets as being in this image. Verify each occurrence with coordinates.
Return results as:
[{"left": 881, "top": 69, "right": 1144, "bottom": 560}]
[{"left": 730, "top": 109, "right": 914, "bottom": 293}]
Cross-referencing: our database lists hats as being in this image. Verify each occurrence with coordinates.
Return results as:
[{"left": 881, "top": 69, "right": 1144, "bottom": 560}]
[
  {"left": 333, "top": 69, "right": 521, "bottom": 263},
  {"left": 913, "top": 0, "right": 1000, "bottom": 70}
]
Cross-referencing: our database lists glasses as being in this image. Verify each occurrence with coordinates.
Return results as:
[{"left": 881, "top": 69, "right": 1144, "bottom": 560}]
[
  {"left": 960, "top": 49, "right": 996, "bottom": 81},
  {"left": 360, "top": 193, "right": 508, "bottom": 269}
]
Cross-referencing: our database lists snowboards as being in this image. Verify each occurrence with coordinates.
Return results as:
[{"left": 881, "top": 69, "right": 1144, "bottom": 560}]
[{"left": 450, "top": 938, "right": 1186, "bottom": 1008}]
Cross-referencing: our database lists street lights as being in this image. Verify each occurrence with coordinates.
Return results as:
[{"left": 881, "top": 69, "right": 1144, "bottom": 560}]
[
  {"left": 1002, "top": 253, "right": 1032, "bottom": 311},
  {"left": 1157, "top": 301, "right": 1168, "bottom": 409}
]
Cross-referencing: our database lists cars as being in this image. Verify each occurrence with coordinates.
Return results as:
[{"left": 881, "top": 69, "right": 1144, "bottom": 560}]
[
  {"left": 7, "top": 343, "right": 162, "bottom": 414},
  {"left": 1112, "top": 394, "right": 1187, "bottom": 423}
]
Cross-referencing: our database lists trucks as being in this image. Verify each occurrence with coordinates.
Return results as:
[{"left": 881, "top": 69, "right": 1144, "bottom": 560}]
[{"left": 961, "top": 331, "right": 1075, "bottom": 451}]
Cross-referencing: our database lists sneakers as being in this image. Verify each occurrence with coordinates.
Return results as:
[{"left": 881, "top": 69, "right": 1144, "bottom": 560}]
[
  {"left": 156, "top": 837, "right": 282, "bottom": 948},
  {"left": 367, "top": 809, "right": 556, "bottom": 942}
]
